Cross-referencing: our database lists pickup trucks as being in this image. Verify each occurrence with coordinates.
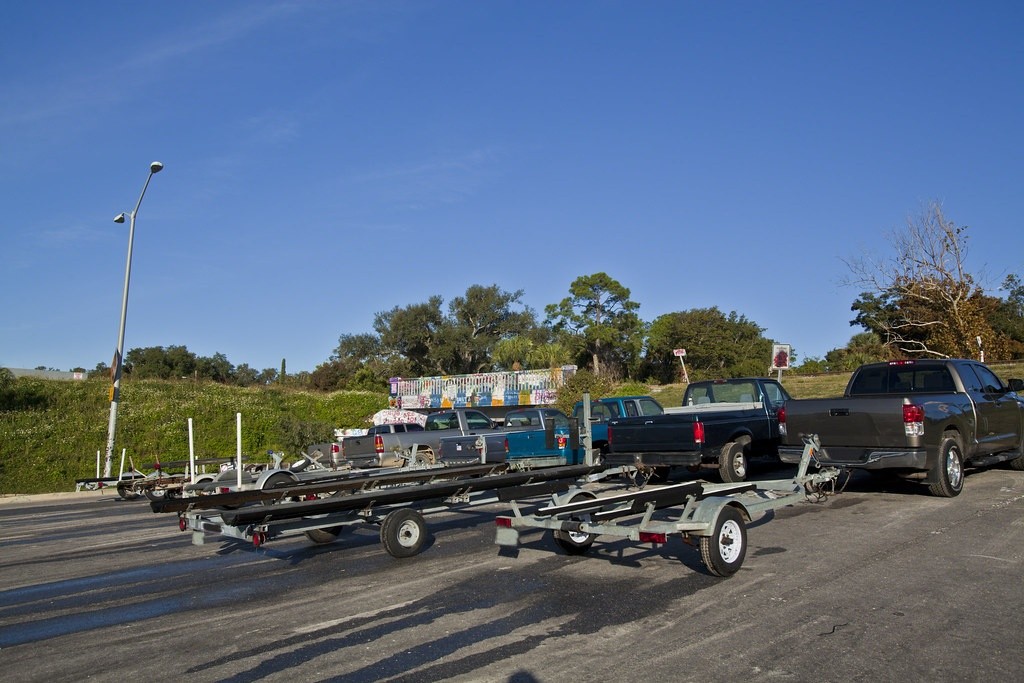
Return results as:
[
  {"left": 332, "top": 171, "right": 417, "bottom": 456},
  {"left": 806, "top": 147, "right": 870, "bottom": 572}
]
[
  {"left": 342, "top": 408, "right": 502, "bottom": 469},
  {"left": 605, "top": 378, "right": 794, "bottom": 483},
  {"left": 307, "top": 422, "right": 424, "bottom": 467},
  {"left": 777, "top": 358, "right": 1024, "bottom": 499},
  {"left": 437, "top": 408, "right": 570, "bottom": 465},
  {"left": 504, "top": 396, "right": 665, "bottom": 472}
]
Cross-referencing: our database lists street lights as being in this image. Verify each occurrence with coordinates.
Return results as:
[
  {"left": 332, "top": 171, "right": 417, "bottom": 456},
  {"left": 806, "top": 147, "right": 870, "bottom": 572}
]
[{"left": 104, "top": 161, "right": 164, "bottom": 478}]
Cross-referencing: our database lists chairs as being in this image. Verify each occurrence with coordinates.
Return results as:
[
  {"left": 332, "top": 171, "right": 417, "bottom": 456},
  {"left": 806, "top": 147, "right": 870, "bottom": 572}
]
[
  {"left": 924, "top": 372, "right": 949, "bottom": 390},
  {"left": 698, "top": 396, "right": 711, "bottom": 404},
  {"left": 882, "top": 374, "right": 911, "bottom": 394},
  {"left": 740, "top": 393, "right": 753, "bottom": 403}
]
